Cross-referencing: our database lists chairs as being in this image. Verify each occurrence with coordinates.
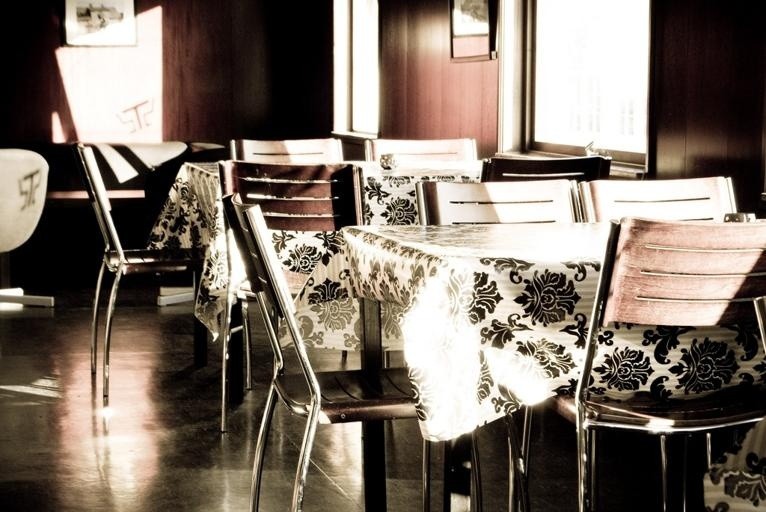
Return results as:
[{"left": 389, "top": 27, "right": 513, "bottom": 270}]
[
  {"left": 74, "top": 132, "right": 766, "bottom": 511},
  {"left": 0, "top": 148, "right": 55, "bottom": 308}
]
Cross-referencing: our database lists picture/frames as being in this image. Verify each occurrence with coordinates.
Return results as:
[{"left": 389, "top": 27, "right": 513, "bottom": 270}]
[{"left": 449, "top": 0, "right": 494, "bottom": 63}]
